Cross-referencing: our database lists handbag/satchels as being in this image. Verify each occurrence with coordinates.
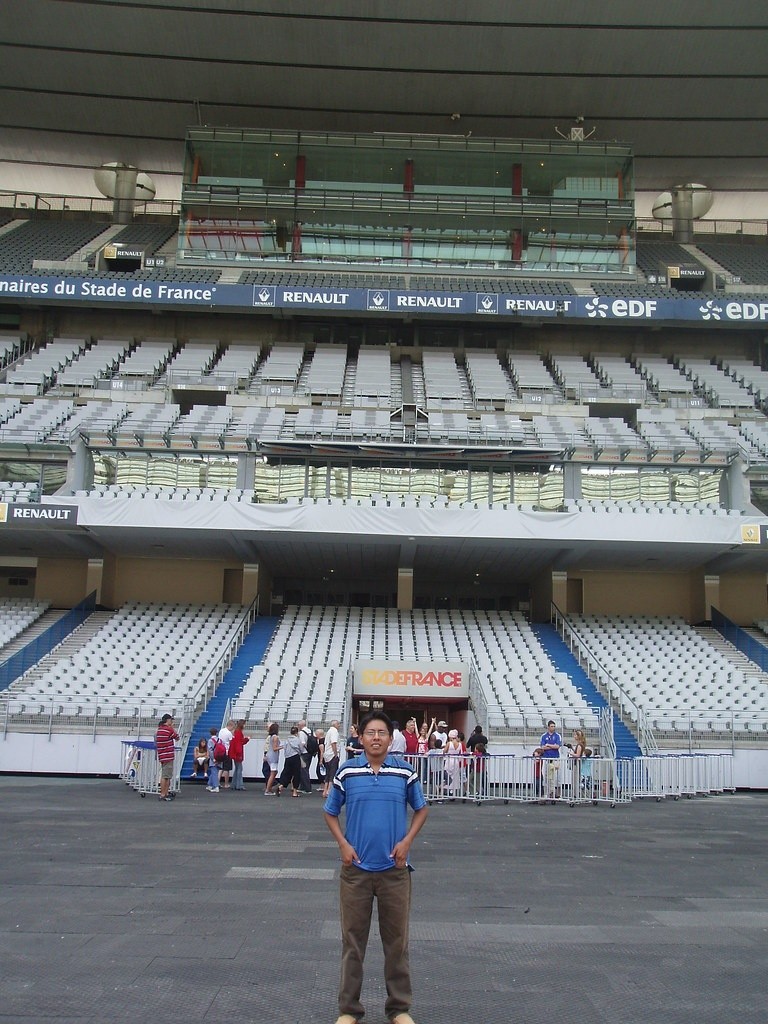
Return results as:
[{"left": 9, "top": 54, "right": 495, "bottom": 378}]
[{"left": 300, "top": 757, "right": 307, "bottom": 768}]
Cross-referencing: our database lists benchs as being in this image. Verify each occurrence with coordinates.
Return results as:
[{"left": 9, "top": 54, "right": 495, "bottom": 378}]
[{"left": 0, "top": 326, "right": 768, "bottom": 446}]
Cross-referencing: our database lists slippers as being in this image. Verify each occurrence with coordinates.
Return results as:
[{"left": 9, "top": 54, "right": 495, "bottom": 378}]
[
  {"left": 293, "top": 795, "right": 300, "bottom": 797},
  {"left": 277, "top": 787, "right": 281, "bottom": 796}
]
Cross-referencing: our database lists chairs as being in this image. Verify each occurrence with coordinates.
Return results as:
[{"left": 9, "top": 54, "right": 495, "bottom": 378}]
[
  {"left": 0, "top": 482, "right": 741, "bottom": 517},
  {"left": 0, "top": 216, "right": 768, "bottom": 302},
  {"left": 0, "top": 598, "right": 768, "bottom": 734}
]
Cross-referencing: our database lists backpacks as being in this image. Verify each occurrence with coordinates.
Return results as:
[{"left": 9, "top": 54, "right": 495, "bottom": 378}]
[
  {"left": 212, "top": 738, "right": 226, "bottom": 760},
  {"left": 301, "top": 729, "right": 319, "bottom": 755}
]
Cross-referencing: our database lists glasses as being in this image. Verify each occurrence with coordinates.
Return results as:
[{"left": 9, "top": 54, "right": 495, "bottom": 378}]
[{"left": 364, "top": 731, "right": 389, "bottom": 736}]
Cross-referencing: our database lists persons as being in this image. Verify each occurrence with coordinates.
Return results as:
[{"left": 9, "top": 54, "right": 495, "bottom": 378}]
[
  {"left": 298, "top": 720, "right": 315, "bottom": 794},
  {"left": 276, "top": 727, "right": 302, "bottom": 797},
  {"left": 531, "top": 721, "right": 562, "bottom": 804},
  {"left": 322, "top": 720, "right": 341, "bottom": 798},
  {"left": 411, "top": 717, "right": 466, "bottom": 801},
  {"left": 387, "top": 721, "right": 407, "bottom": 759},
  {"left": 218, "top": 720, "right": 236, "bottom": 788},
  {"left": 315, "top": 729, "right": 326, "bottom": 791},
  {"left": 402, "top": 720, "right": 419, "bottom": 765},
  {"left": 153, "top": 714, "right": 180, "bottom": 801},
  {"left": 228, "top": 719, "right": 251, "bottom": 790},
  {"left": 262, "top": 724, "right": 283, "bottom": 796},
  {"left": 568, "top": 730, "right": 596, "bottom": 804},
  {"left": 191, "top": 739, "right": 209, "bottom": 778},
  {"left": 206, "top": 728, "right": 222, "bottom": 792},
  {"left": 345, "top": 724, "right": 364, "bottom": 760},
  {"left": 323, "top": 710, "right": 429, "bottom": 1024},
  {"left": 466, "top": 726, "right": 489, "bottom": 799}
]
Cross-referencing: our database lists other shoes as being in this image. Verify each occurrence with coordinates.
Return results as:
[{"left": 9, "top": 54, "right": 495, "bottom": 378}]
[
  {"left": 336, "top": 1015, "right": 357, "bottom": 1024},
  {"left": 190, "top": 773, "right": 197, "bottom": 776},
  {"left": 236, "top": 787, "right": 246, "bottom": 790},
  {"left": 323, "top": 794, "right": 329, "bottom": 797},
  {"left": 297, "top": 790, "right": 303, "bottom": 792},
  {"left": 393, "top": 1013, "right": 415, "bottom": 1024},
  {"left": 265, "top": 792, "right": 276, "bottom": 795},
  {"left": 303, "top": 791, "right": 312, "bottom": 794},
  {"left": 225, "top": 784, "right": 230, "bottom": 788},
  {"left": 210, "top": 788, "right": 219, "bottom": 792},
  {"left": 204, "top": 773, "right": 208, "bottom": 777},
  {"left": 206, "top": 786, "right": 213, "bottom": 790},
  {"left": 160, "top": 795, "right": 174, "bottom": 801}
]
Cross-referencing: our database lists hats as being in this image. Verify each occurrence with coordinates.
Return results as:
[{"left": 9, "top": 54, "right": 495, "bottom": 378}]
[
  {"left": 163, "top": 714, "right": 175, "bottom": 720},
  {"left": 438, "top": 721, "right": 448, "bottom": 727}
]
